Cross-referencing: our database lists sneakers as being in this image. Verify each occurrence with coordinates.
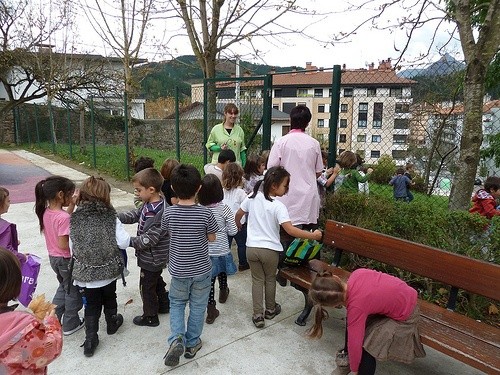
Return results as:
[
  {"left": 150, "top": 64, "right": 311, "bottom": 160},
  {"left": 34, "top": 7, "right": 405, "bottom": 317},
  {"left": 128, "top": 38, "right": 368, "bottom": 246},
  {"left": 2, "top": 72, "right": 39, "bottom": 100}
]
[
  {"left": 264, "top": 303, "right": 281, "bottom": 319},
  {"left": 158, "top": 304, "right": 169, "bottom": 313},
  {"left": 252, "top": 316, "right": 264, "bottom": 326},
  {"left": 133, "top": 314, "right": 159, "bottom": 326},
  {"left": 163, "top": 339, "right": 184, "bottom": 366},
  {"left": 184, "top": 338, "right": 202, "bottom": 358}
]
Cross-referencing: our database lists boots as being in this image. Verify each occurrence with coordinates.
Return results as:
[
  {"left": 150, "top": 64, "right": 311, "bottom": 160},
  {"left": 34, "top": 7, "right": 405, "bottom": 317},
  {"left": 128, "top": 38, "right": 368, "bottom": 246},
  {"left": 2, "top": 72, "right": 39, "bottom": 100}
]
[
  {"left": 206, "top": 301, "right": 219, "bottom": 323},
  {"left": 80, "top": 316, "right": 99, "bottom": 356},
  {"left": 104, "top": 308, "right": 123, "bottom": 335},
  {"left": 219, "top": 287, "right": 230, "bottom": 303}
]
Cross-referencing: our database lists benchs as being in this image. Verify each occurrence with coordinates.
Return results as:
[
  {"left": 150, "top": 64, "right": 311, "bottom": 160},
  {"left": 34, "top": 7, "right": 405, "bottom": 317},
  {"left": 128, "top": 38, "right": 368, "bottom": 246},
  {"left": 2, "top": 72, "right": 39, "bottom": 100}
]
[{"left": 279, "top": 220, "right": 500, "bottom": 375}]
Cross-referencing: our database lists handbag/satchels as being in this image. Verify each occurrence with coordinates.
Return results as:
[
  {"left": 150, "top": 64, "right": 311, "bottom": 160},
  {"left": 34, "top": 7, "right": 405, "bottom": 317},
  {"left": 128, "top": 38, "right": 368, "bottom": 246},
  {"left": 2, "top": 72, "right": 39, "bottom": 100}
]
[
  {"left": 279, "top": 237, "right": 323, "bottom": 267},
  {"left": 18, "top": 253, "right": 43, "bottom": 308}
]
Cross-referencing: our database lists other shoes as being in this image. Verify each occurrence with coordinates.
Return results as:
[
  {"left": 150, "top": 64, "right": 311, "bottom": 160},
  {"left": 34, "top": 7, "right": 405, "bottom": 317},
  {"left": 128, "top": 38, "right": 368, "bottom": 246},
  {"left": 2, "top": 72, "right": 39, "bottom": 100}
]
[
  {"left": 62, "top": 317, "right": 84, "bottom": 335},
  {"left": 239, "top": 264, "right": 249, "bottom": 271}
]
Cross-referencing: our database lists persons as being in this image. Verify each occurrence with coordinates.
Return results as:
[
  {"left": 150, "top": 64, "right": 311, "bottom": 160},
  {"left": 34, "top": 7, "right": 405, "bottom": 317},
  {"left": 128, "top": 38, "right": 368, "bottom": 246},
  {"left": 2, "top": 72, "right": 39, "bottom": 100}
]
[
  {"left": 161, "top": 158, "right": 181, "bottom": 207},
  {"left": 206, "top": 103, "right": 247, "bottom": 168},
  {"left": 468, "top": 176, "right": 500, "bottom": 232},
  {"left": 234, "top": 166, "right": 322, "bottom": 328},
  {"left": 304, "top": 267, "right": 426, "bottom": 375},
  {"left": 388, "top": 163, "right": 415, "bottom": 204},
  {"left": 198, "top": 173, "right": 238, "bottom": 324},
  {"left": 160, "top": 163, "right": 219, "bottom": 366},
  {"left": 66, "top": 176, "right": 130, "bottom": 356},
  {"left": 0, "top": 187, "right": 63, "bottom": 375},
  {"left": 204, "top": 145, "right": 374, "bottom": 270},
  {"left": 34, "top": 177, "right": 86, "bottom": 335},
  {"left": 268, "top": 104, "right": 323, "bottom": 287},
  {"left": 115, "top": 168, "right": 171, "bottom": 327},
  {"left": 133, "top": 156, "right": 155, "bottom": 208}
]
